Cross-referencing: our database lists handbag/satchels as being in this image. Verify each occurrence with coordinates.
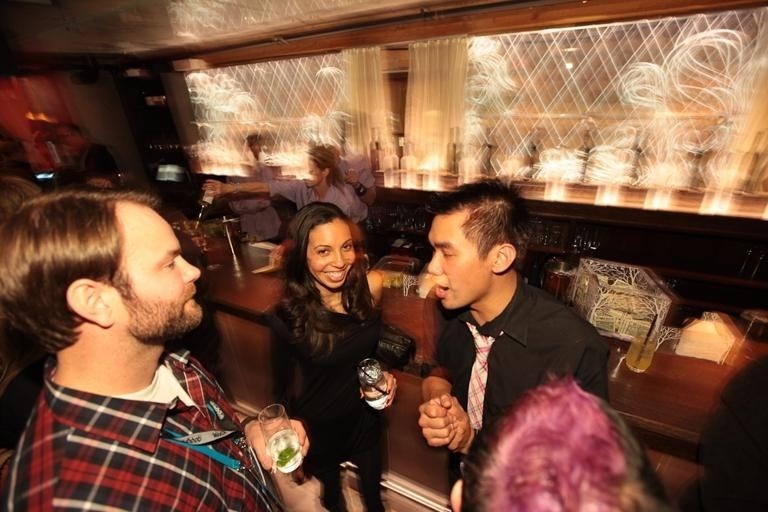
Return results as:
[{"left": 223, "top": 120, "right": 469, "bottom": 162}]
[{"left": 377, "top": 324, "right": 414, "bottom": 367}]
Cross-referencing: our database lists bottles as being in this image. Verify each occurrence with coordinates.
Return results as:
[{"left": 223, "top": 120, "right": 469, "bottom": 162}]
[
  {"left": 357, "top": 358, "right": 388, "bottom": 411},
  {"left": 198, "top": 189, "right": 214, "bottom": 213}
]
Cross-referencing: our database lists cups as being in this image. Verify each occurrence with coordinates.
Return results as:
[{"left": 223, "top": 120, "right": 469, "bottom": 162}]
[
  {"left": 624, "top": 327, "right": 658, "bottom": 373},
  {"left": 257, "top": 403, "right": 303, "bottom": 474}
]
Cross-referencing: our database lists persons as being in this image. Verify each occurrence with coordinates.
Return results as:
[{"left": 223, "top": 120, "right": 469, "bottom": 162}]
[
  {"left": 0, "top": 120, "right": 397, "bottom": 511},
  {"left": 451, "top": 374, "right": 669, "bottom": 512},
  {"left": 679, "top": 352, "right": 768, "bottom": 512},
  {"left": 417, "top": 176, "right": 610, "bottom": 512}
]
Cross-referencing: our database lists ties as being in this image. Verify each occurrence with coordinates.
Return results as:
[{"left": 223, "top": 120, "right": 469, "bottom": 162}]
[{"left": 462, "top": 322, "right": 504, "bottom": 431}]
